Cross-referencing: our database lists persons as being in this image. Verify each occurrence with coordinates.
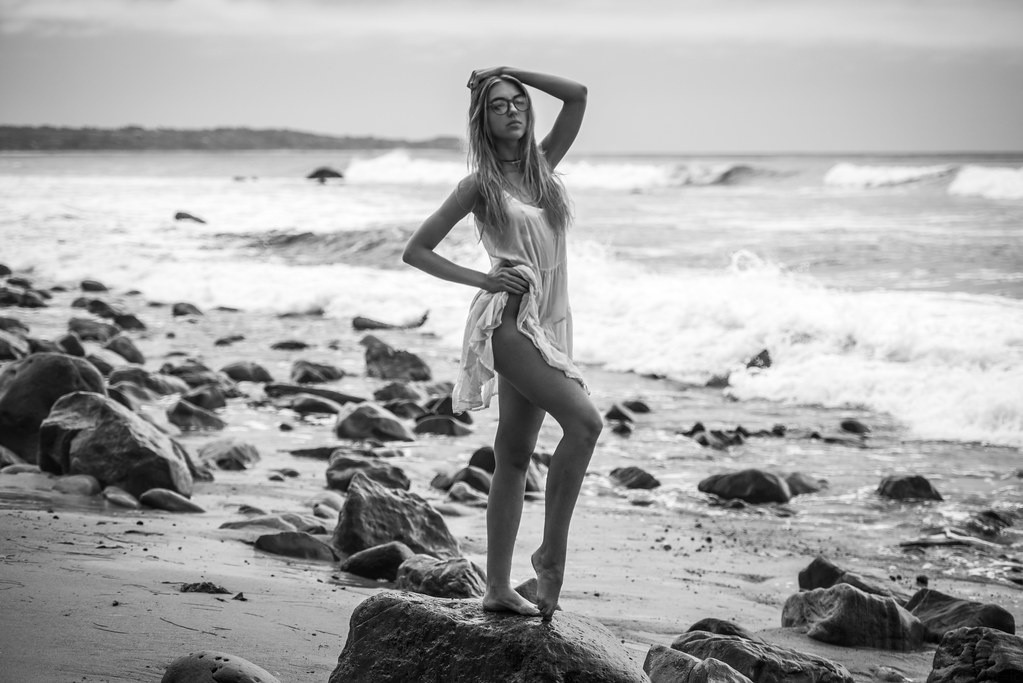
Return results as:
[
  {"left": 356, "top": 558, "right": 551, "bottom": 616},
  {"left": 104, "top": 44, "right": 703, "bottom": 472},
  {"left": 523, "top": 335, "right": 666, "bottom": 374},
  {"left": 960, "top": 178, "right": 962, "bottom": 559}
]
[{"left": 402, "top": 67, "right": 603, "bottom": 622}]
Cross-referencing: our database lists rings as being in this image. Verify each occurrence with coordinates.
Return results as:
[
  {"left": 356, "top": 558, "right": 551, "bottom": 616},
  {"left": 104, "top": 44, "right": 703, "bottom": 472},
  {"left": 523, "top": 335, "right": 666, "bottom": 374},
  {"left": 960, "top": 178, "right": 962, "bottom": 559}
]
[{"left": 470, "top": 82, "right": 473, "bottom": 85}]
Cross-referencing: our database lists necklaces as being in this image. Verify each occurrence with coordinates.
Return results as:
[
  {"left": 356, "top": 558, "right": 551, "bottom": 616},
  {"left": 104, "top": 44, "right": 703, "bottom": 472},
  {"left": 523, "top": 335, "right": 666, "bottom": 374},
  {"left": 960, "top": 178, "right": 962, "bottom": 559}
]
[{"left": 502, "top": 159, "right": 521, "bottom": 164}]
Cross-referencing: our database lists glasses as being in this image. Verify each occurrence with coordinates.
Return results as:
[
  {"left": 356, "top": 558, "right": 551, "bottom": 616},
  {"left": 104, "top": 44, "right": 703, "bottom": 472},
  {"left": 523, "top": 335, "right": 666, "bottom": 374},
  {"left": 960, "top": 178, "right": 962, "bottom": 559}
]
[{"left": 487, "top": 93, "right": 530, "bottom": 115}]
